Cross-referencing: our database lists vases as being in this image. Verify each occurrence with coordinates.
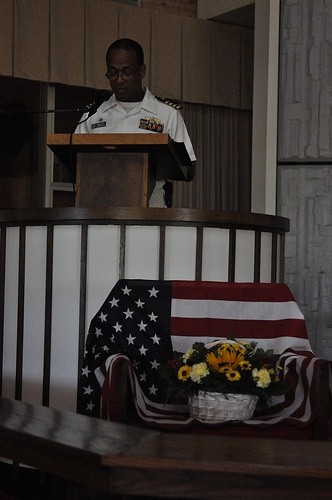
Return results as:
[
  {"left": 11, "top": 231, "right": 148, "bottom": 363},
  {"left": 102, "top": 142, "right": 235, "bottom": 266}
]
[{"left": 187, "top": 389, "right": 258, "bottom": 423}]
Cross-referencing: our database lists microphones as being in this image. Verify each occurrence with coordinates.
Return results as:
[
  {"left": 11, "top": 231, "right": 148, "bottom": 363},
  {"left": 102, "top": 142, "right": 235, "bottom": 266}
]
[{"left": 88, "top": 91, "right": 114, "bottom": 116}]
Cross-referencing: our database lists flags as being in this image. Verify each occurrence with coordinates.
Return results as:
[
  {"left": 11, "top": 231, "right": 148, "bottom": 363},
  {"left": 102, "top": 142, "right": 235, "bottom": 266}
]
[{"left": 76, "top": 277, "right": 332, "bottom": 430}]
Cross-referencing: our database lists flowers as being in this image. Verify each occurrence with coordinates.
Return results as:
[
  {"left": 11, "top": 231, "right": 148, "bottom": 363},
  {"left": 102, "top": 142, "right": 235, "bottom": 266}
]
[{"left": 177, "top": 338, "right": 284, "bottom": 397}]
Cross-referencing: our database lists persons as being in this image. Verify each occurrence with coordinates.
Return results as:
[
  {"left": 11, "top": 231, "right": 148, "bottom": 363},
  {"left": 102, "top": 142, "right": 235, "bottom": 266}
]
[{"left": 72, "top": 38, "right": 199, "bottom": 208}]
